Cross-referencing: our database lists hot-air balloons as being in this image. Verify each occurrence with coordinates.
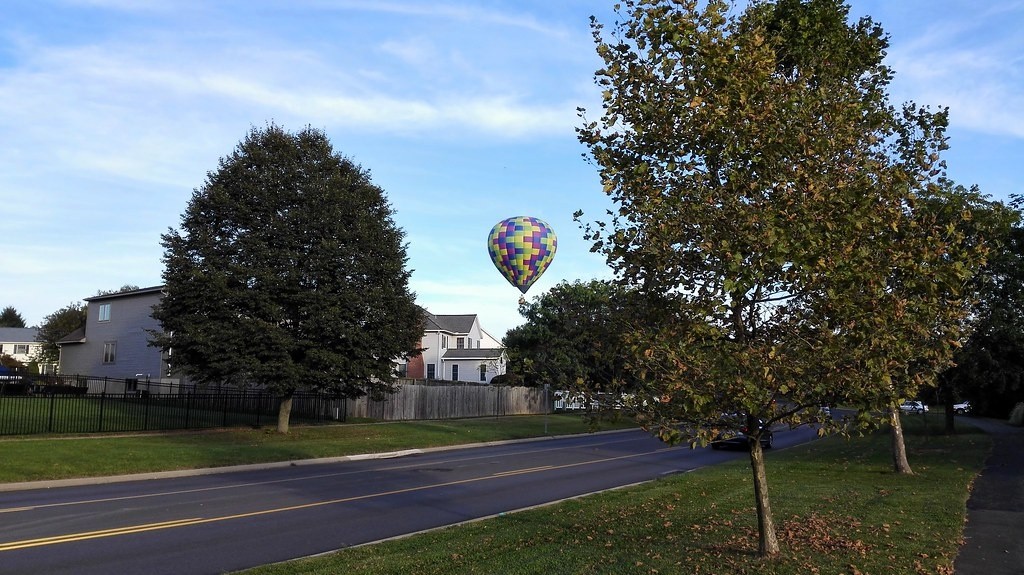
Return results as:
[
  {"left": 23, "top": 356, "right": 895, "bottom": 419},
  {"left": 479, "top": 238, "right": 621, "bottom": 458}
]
[{"left": 488, "top": 216, "right": 558, "bottom": 304}]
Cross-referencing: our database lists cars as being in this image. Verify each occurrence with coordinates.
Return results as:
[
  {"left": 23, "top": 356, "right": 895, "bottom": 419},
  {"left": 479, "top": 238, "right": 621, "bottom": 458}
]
[
  {"left": 898, "top": 400, "right": 930, "bottom": 415},
  {"left": 710, "top": 413, "right": 773, "bottom": 452},
  {"left": 952, "top": 399, "right": 976, "bottom": 416},
  {"left": 816, "top": 406, "right": 831, "bottom": 423}
]
